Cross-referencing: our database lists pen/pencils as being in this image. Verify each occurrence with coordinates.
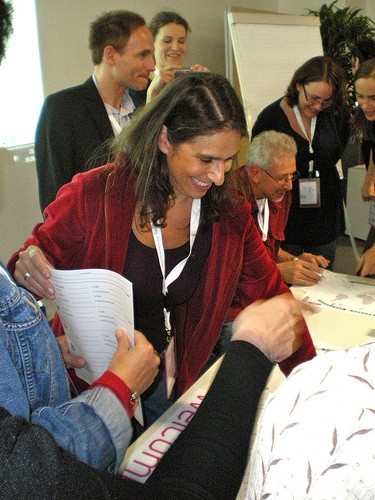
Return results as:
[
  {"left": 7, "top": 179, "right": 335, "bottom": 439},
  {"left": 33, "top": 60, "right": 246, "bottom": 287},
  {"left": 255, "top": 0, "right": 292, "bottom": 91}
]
[{"left": 291, "top": 256, "right": 328, "bottom": 280}]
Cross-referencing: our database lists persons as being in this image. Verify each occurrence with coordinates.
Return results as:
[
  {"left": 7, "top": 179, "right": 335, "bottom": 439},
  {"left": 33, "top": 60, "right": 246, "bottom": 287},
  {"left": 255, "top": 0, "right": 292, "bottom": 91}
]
[
  {"left": 251, "top": 57, "right": 346, "bottom": 270},
  {"left": 148, "top": 11, "right": 211, "bottom": 101},
  {"left": 1, "top": 294, "right": 303, "bottom": 499},
  {"left": 242, "top": 132, "right": 329, "bottom": 287},
  {"left": 0, "top": 262, "right": 160, "bottom": 473},
  {"left": 6, "top": 72, "right": 318, "bottom": 422},
  {"left": 353, "top": 57, "right": 375, "bottom": 277},
  {"left": 35, "top": 11, "right": 156, "bottom": 214}
]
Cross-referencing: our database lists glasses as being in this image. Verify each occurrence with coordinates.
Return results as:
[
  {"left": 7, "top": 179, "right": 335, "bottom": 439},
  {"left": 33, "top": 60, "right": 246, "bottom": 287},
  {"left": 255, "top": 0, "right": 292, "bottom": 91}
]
[
  {"left": 301, "top": 83, "right": 332, "bottom": 108},
  {"left": 259, "top": 167, "right": 301, "bottom": 183}
]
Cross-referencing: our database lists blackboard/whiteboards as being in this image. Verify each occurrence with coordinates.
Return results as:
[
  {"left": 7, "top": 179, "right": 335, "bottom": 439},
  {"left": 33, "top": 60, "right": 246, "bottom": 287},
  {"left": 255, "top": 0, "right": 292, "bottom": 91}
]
[{"left": 225, "top": 5, "right": 325, "bottom": 143}]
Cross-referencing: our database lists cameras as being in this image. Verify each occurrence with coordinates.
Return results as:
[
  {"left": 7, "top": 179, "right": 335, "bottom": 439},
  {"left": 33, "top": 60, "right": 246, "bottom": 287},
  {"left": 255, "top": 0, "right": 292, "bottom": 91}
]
[{"left": 173, "top": 68, "right": 191, "bottom": 79}]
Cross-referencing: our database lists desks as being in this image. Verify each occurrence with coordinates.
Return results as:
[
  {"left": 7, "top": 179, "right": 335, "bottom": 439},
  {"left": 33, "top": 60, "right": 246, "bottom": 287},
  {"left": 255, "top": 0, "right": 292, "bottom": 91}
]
[{"left": 118, "top": 270, "right": 375, "bottom": 500}]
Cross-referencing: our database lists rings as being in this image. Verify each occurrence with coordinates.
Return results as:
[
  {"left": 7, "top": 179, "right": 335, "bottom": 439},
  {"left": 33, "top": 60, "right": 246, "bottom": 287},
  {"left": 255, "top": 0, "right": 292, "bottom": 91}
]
[{"left": 24, "top": 271, "right": 32, "bottom": 281}]
